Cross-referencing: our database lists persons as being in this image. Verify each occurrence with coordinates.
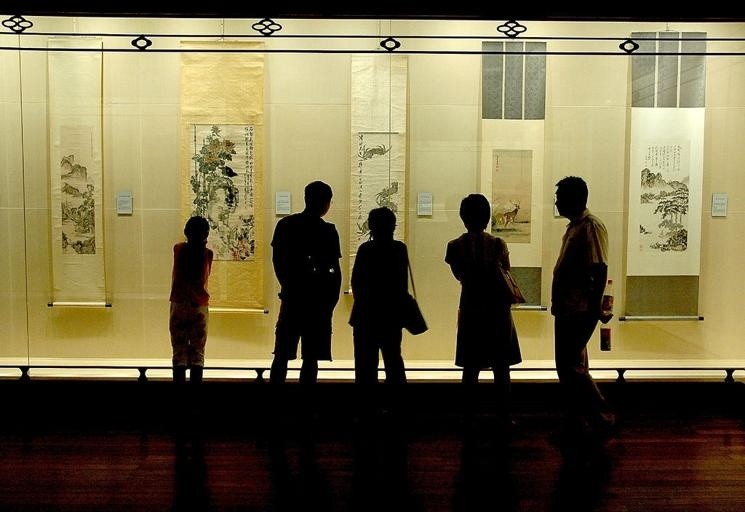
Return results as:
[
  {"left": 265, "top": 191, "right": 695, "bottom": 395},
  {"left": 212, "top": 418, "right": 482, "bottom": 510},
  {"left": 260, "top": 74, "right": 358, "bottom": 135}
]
[
  {"left": 349, "top": 207, "right": 427, "bottom": 383},
  {"left": 551, "top": 178, "right": 621, "bottom": 446},
  {"left": 445, "top": 196, "right": 525, "bottom": 435},
  {"left": 267, "top": 183, "right": 341, "bottom": 383},
  {"left": 170, "top": 217, "right": 213, "bottom": 382}
]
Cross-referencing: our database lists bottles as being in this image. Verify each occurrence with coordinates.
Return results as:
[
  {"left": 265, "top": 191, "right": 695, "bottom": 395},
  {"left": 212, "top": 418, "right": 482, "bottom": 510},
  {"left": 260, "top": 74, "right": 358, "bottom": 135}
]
[
  {"left": 602, "top": 280, "right": 615, "bottom": 315},
  {"left": 600, "top": 322, "right": 613, "bottom": 352}
]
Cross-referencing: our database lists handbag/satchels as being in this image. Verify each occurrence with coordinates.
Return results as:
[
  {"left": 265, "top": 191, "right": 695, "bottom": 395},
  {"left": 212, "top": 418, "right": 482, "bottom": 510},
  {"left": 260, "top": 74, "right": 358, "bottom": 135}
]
[
  {"left": 394, "top": 288, "right": 429, "bottom": 335},
  {"left": 547, "top": 262, "right": 607, "bottom": 319},
  {"left": 492, "top": 234, "right": 529, "bottom": 305}
]
